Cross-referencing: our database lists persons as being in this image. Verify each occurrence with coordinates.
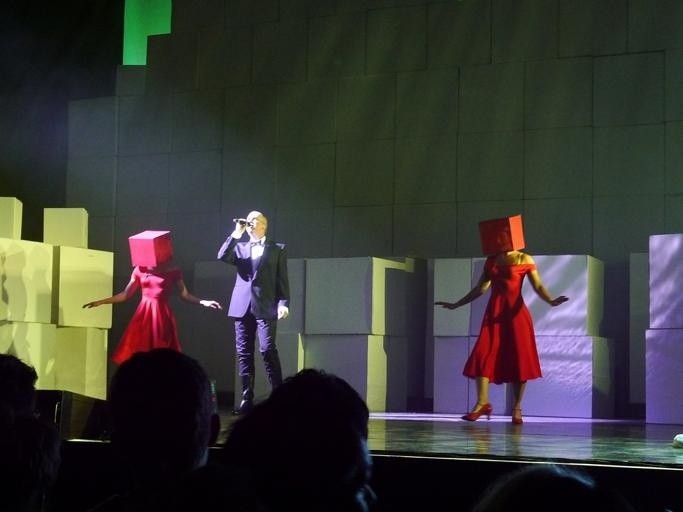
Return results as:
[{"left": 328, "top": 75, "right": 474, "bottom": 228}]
[
  {"left": 0, "top": 420, "right": 59, "bottom": 510},
  {"left": 0, "top": 352, "right": 37, "bottom": 423},
  {"left": 215, "top": 210, "right": 291, "bottom": 417},
  {"left": 221, "top": 392, "right": 379, "bottom": 512},
  {"left": 81, "top": 230, "right": 223, "bottom": 367},
  {"left": 98, "top": 345, "right": 222, "bottom": 511},
  {"left": 433, "top": 214, "right": 569, "bottom": 426},
  {"left": 269, "top": 368, "right": 369, "bottom": 444}
]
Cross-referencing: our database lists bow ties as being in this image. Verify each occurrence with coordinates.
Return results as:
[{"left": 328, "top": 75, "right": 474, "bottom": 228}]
[{"left": 249, "top": 240, "right": 260, "bottom": 248}]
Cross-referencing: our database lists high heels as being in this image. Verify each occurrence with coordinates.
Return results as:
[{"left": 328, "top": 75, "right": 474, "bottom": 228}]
[
  {"left": 512, "top": 409, "right": 523, "bottom": 424},
  {"left": 462, "top": 403, "right": 493, "bottom": 421}
]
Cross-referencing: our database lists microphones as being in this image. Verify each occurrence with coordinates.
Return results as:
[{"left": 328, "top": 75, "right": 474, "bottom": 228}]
[{"left": 233, "top": 218, "right": 250, "bottom": 227}]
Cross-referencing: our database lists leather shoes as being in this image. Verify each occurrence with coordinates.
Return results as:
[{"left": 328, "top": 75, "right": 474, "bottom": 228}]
[{"left": 231, "top": 399, "right": 252, "bottom": 415}]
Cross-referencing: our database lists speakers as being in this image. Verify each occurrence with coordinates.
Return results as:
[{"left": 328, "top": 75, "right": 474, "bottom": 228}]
[{"left": 34, "top": 389, "right": 110, "bottom": 440}]
